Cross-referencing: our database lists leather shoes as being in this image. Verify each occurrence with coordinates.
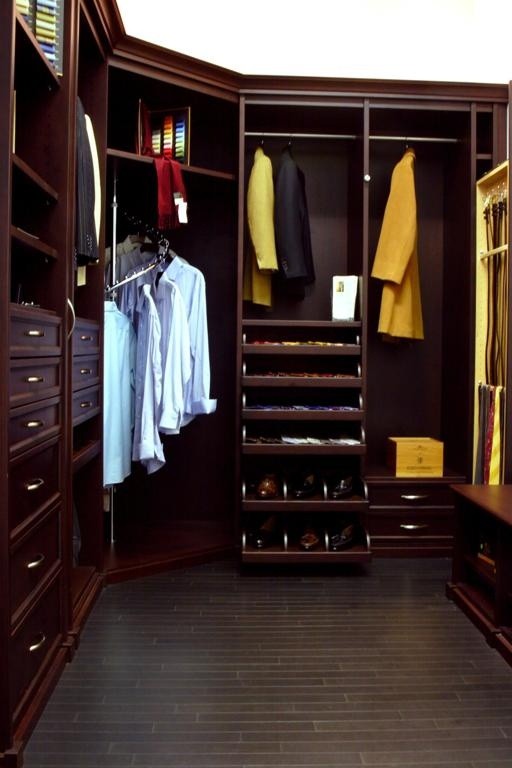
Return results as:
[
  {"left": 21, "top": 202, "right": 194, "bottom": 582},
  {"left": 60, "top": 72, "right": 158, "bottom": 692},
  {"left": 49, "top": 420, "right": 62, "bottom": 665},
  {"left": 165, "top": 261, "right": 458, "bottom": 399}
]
[{"left": 255, "top": 473, "right": 354, "bottom": 551}]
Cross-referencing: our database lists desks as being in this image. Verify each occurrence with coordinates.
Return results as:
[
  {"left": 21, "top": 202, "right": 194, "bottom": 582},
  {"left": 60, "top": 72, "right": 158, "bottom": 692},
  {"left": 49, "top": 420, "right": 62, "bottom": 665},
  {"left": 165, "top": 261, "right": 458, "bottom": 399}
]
[{"left": 444, "top": 476, "right": 511, "bottom": 662}]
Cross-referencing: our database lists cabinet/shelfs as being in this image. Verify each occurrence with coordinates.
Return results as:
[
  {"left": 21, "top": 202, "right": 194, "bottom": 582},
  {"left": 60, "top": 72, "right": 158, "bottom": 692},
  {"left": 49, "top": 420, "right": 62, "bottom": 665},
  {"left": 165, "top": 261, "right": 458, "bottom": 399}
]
[
  {"left": 0, "top": 0, "right": 67, "bottom": 767},
  {"left": 370, "top": 77, "right": 477, "bottom": 555},
  {"left": 65, "top": 1, "right": 108, "bottom": 653},
  {"left": 474, "top": 156, "right": 512, "bottom": 488},
  {"left": 106, "top": 32, "right": 246, "bottom": 179},
  {"left": 241, "top": 74, "right": 371, "bottom": 573}
]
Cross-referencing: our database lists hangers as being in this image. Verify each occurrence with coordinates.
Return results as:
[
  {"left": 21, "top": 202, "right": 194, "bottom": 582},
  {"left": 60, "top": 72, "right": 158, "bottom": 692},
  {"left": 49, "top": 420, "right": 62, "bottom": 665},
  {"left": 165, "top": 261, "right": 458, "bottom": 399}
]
[{"left": 101, "top": 224, "right": 204, "bottom": 316}]
